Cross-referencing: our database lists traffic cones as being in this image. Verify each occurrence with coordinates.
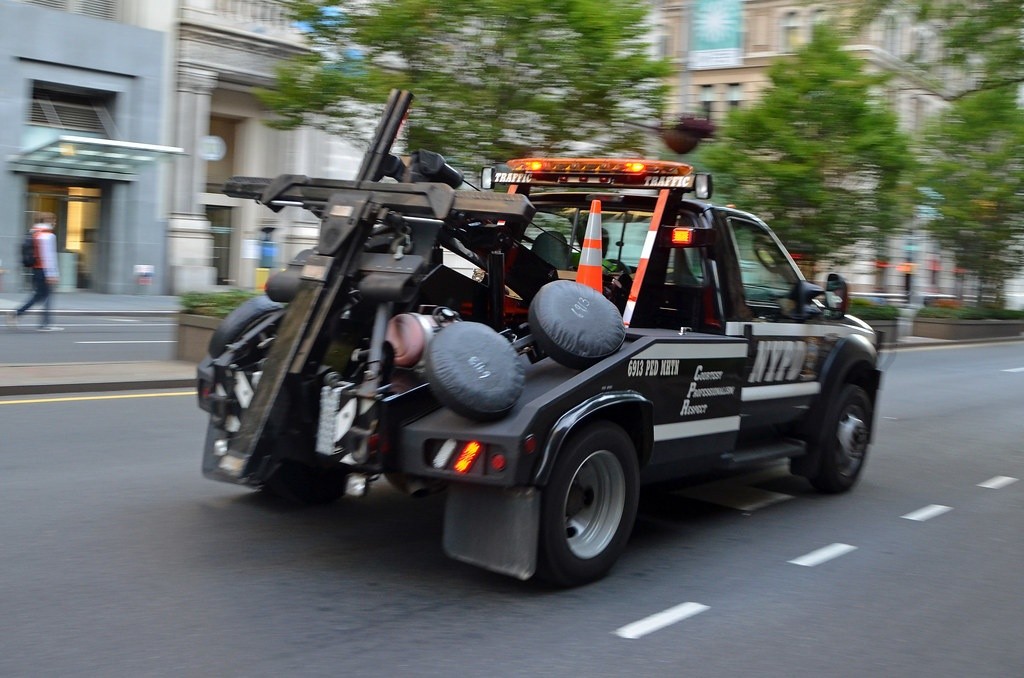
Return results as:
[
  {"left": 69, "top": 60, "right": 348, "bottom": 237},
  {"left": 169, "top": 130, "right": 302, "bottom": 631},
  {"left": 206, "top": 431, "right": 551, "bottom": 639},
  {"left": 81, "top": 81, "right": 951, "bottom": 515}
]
[{"left": 574, "top": 198, "right": 603, "bottom": 295}]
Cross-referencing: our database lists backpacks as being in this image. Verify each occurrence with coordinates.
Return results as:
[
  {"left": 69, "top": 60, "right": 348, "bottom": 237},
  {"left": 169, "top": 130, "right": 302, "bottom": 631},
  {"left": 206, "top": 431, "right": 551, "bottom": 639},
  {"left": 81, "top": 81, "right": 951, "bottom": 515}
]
[{"left": 21, "top": 225, "right": 55, "bottom": 268}]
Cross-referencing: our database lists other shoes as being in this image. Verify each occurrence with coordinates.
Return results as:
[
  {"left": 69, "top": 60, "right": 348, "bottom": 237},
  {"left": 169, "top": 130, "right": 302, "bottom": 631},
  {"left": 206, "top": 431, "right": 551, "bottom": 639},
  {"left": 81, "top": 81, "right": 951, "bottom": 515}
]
[
  {"left": 34, "top": 323, "right": 65, "bottom": 333},
  {"left": 6, "top": 308, "right": 19, "bottom": 330}
]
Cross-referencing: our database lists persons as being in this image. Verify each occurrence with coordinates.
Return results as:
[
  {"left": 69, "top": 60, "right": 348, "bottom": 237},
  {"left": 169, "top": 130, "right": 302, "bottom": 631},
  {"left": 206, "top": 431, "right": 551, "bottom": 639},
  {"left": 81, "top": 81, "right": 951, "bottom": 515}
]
[
  {"left": 9, "top": 213, "right": 65, "bottom": 332},
  {"left": 572, "top": 228, "right": 619, "bottom": 272}
]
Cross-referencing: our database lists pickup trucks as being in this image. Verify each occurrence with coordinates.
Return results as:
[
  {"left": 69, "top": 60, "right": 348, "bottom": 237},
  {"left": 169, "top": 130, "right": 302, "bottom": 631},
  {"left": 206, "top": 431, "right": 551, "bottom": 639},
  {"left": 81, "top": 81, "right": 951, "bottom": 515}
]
[{"left": 195, "top": 155, "right": 886, "bottom": 592}]
